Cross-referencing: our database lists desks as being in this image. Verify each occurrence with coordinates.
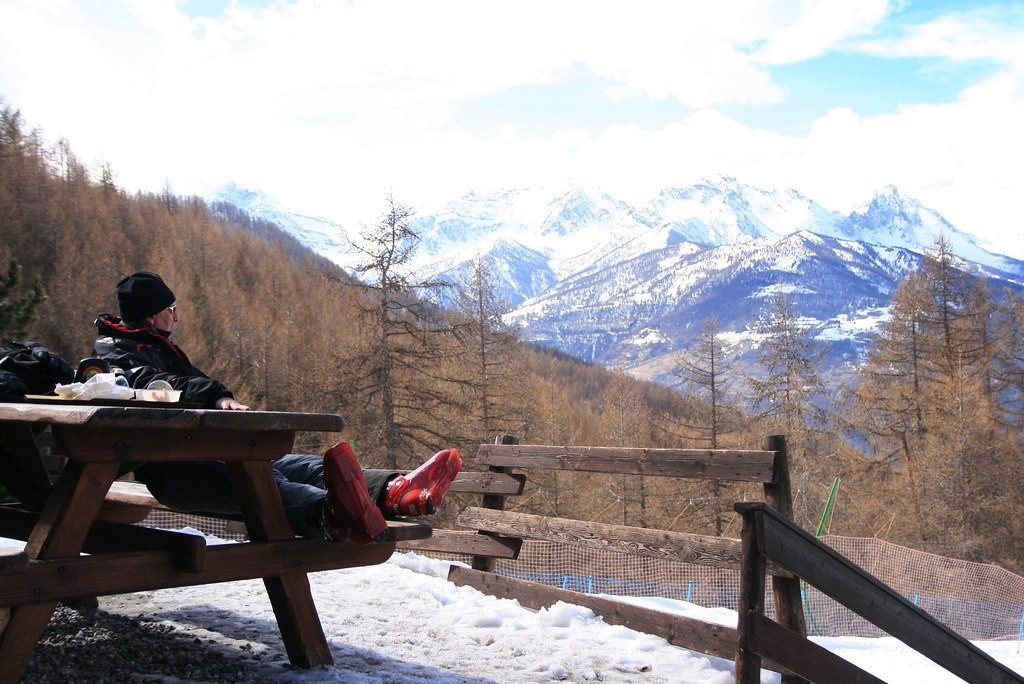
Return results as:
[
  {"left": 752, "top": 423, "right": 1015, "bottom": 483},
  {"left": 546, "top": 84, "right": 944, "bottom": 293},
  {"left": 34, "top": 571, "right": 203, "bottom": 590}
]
[{"left": 0, "top": 403, "right": 345, "bottom": 684}]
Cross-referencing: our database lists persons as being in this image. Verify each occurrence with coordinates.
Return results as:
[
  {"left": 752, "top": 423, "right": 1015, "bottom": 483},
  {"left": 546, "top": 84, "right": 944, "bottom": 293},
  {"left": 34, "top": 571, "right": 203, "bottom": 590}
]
[{"left": 90, "top": 271, "right": 462, "bottom": 545}]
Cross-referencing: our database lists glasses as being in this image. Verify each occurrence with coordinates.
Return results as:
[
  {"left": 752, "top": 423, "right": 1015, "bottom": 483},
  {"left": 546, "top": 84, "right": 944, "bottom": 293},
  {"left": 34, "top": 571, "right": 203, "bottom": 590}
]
[{"left": 165, "top": 304, "right": 177, "bottom": 315}]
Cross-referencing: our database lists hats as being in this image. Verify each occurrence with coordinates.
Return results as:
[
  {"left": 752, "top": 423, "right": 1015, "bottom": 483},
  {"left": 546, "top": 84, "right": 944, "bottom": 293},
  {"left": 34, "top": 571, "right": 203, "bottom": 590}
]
[{"left": 116, "top": 271, "right": 177, "bottom": 324}]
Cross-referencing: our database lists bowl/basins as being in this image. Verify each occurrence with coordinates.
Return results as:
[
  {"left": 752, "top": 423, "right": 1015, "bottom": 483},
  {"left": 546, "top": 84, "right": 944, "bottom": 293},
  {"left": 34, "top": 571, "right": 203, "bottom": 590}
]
[{"left": 134, "top": 388, "right": 182, "bottom": 402}]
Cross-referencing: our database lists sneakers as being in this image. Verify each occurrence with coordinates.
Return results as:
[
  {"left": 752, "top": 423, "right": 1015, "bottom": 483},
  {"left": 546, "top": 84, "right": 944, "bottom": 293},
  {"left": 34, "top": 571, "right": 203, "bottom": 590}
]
[
  {"left": 384, "top": 448, "right": 461, "bottom": 517},
  {"left": 321, "top": 441, "right": 389, "bottom": 547}
]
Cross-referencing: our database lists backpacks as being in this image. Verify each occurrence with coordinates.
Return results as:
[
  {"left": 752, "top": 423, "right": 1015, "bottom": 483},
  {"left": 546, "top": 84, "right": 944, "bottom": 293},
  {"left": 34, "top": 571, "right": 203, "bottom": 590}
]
[{"left": 0, "top": 341, "right": 75, "bottom": 396}]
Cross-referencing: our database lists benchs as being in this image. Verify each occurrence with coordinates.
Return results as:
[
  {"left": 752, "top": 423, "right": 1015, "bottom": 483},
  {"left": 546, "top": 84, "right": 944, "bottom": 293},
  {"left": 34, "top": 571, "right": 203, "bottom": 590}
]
[{"left": 0, "top": 480, "right": 433, "bottom": 684}]
